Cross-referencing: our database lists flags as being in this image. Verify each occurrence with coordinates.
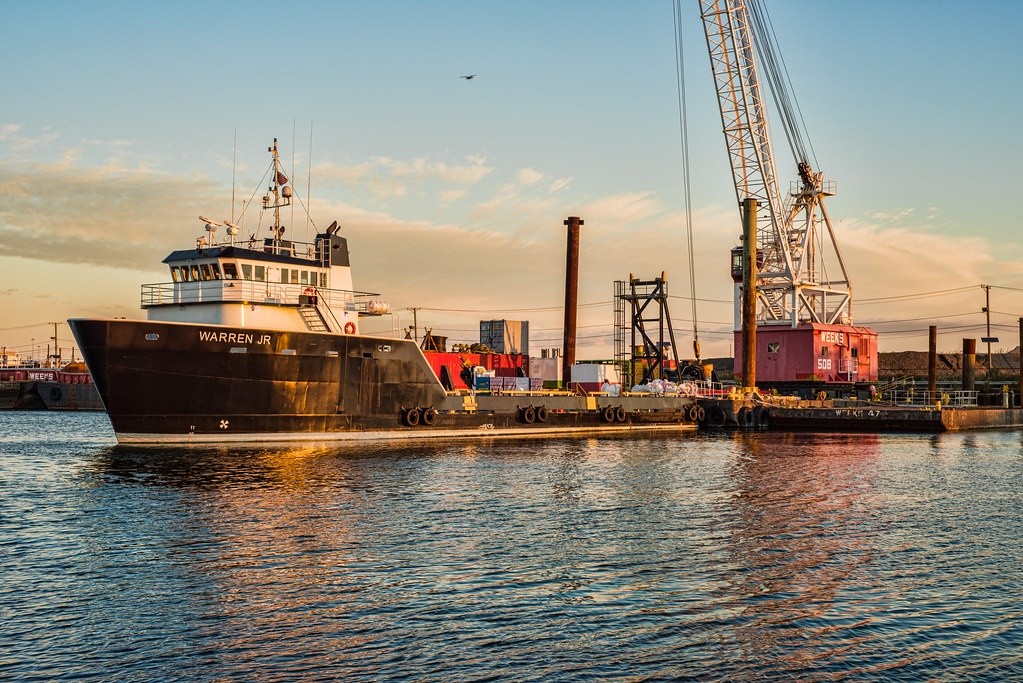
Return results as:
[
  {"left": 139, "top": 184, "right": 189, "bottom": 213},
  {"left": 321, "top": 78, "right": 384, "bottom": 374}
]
[{"left": 272, "top": 170, "right": 288, "bottom": 185}]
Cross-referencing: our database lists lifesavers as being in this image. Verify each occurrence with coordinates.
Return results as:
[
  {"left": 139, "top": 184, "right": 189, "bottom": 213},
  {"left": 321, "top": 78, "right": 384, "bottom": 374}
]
[
  {"left": 304, "top": 288, "right": 318, "bottom": 307},
  {"left": 345, "top": 322, "right": 356, "bottom": 334},
  {"left": 820, "top": 391, "right": 826, "bottom": 400}
]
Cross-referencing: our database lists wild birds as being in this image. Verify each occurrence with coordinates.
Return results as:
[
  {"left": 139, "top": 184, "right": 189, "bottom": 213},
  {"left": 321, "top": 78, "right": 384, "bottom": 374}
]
[{"left": 459, "top": 75, "right": 476, "bottom": 80}]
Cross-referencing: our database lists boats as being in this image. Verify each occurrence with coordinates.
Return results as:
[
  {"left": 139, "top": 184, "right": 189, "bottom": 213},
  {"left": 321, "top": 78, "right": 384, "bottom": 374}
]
[
  {"left": 0, "top": 342, "right": 64, "bottom": 411},
  {"left": 64, "top": 116, "right": 708, "bottom": 440},
  {"left": 27, "top": 345, "right": 104, "bottom": 411}
]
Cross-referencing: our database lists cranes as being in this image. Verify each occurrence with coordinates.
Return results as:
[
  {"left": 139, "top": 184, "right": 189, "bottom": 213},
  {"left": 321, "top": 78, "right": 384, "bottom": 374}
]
[{"left": 672, "top": 0, "right": 852, "bottom": 332}]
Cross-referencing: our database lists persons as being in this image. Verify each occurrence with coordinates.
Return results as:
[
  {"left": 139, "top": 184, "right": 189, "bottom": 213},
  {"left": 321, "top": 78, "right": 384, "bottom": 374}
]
[{"left": 600, "top": 378, "right": 610, "bottom": 397}]
